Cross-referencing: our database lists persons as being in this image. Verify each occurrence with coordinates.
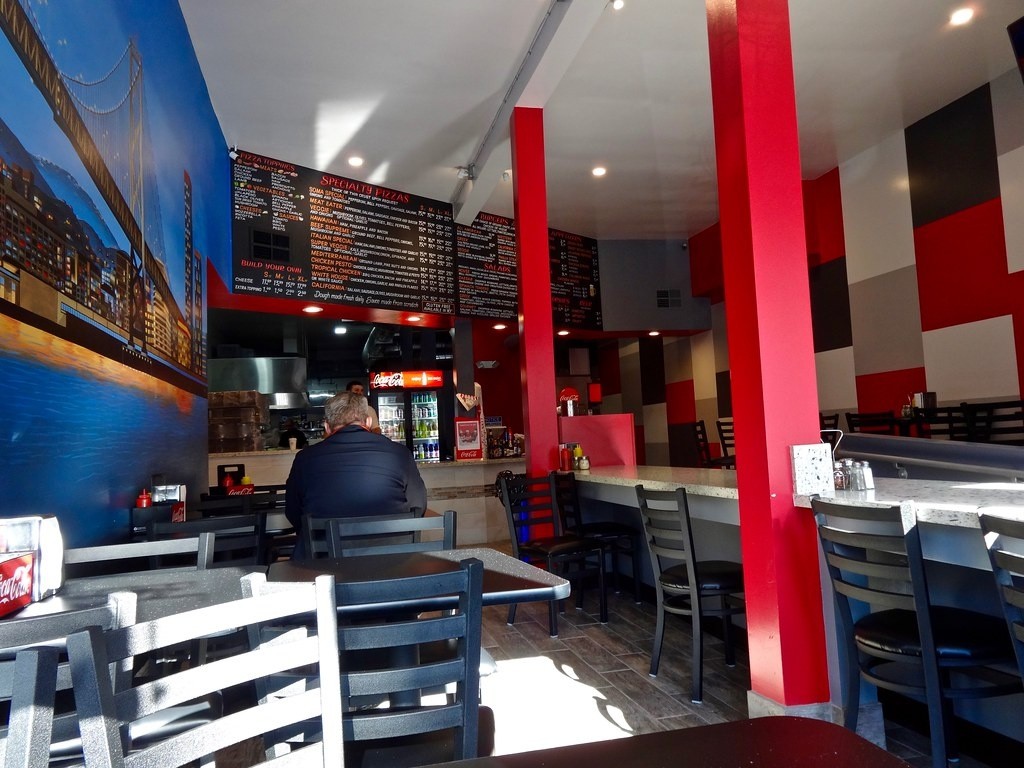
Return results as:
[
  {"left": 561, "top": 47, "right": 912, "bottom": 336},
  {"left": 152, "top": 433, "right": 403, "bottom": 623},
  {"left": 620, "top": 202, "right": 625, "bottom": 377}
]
[
  {"left": 345, "top": 381, "right": 381, "bottom": 435},
  {"left": 277, "top": 419, "right": 307, "bottom": 448},
  {"left": 283, "top": 390, "right": 428, "bottom": 559}
]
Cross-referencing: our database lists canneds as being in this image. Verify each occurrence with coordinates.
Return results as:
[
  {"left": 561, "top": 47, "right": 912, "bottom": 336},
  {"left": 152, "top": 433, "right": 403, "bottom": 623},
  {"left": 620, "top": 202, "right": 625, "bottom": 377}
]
[
  {"left": 412, "top": 407, "right": 437, "bottom": 418},
  {"left": 398, "top": 409, "right": 403, "bottom": 419},
  {"left": 411, "top": 392, "right": 437, "bottom": 402}
]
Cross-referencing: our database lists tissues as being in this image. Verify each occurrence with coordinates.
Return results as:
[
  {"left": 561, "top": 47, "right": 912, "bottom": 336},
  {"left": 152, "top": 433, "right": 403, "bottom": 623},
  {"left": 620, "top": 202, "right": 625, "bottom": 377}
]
[{"left": 0, "top": 514, "right": 67, "bottom": 602}]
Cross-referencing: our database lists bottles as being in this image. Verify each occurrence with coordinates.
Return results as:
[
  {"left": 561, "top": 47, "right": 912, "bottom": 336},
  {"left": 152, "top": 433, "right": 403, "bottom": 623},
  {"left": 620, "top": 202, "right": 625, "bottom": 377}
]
[
  {"left": 222, "top": 473, "right": 234, "bottom": 487},
  {"left": 559, "top": 443, "right": 589, "bottom": 471},
  {"left": 378, "top": 393, "right": 440, "bottom": 459},
  {"left": 487, "top": 429, "right": 522, "bottom": 458},
  {"left": 902, "top": 404, "right": 912, "bottom": 417},
  {"left": 135, "top": 488, "right": 152, "bottom": 508},
  {"left": 832, "top": 458, "right": 875, "bottom": 491},
  {"left": 241, "top": 475, "right": 251, "bottom": 485}
]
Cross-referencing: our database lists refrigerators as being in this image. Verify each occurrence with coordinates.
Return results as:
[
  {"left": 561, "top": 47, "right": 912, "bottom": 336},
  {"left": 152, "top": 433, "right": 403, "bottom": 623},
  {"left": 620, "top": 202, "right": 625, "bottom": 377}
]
[{"left": 366, "top": 368, "right": 445, "bottom": 462}]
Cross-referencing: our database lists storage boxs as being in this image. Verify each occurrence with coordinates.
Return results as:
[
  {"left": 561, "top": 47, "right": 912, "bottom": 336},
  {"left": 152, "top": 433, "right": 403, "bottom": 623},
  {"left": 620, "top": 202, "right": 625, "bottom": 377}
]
[
  {"left": 131, "top": 502, "right": 183, "bottom": 535},
  {"left": 0, "top": 550, "right": 37, "bottom": 619},
  {"left": 209, "top": 485, "right": 255, "bottom": 495},
  {"left": 914, "top": 391, "right": 938, "bottom": 410},
  {"left": 791, "top": 442, "right": 835, "bottom": 495},
  {"left": 560, "top": 441, "right": 579, "bottom": 467}
]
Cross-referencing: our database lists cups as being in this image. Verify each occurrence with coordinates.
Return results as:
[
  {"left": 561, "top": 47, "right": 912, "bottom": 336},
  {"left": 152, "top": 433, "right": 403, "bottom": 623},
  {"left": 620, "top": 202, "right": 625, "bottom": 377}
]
[{"left": 288, "top": 438, "right": 297, "bottom": 449}]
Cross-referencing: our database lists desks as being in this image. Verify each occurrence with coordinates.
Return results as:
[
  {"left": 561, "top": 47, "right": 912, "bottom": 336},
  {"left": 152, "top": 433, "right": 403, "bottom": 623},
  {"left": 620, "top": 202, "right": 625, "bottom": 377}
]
[
  {"left": 1, "top": 547, "right": 571, "bottom": 712},
  {"left": 900, "top": 395, "right": 1024, "bottom": 443},
  {"left": 423, "top": 713, "right": 912, "bottom": 768},
  {"left": 559, "top": 464, "right": 739, "bottom": 528},
  {"left": 792, "top": 473, "right": 1024, "bottom": 555},
  {"left": 64, "top": 510, "right": 441, "bottom": 568}
]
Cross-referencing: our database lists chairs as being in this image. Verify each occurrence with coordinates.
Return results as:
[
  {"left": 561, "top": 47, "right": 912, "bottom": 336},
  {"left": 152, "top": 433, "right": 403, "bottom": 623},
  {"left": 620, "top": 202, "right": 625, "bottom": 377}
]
[
  {"left": 692, "top": 420, "right": 739, "bottom": 474},
  {"left": 817, "top": 401, "right": 1024, "bottom": 445},
  {"left": 0, "top": 485, "right": 485, "bottom": 768},
  {"left": 634, "top": 484, "right": 746, "bottom": 704},
  {"left": 809, "top": 495, "right": 1024, "bottom": 768},
  {"left": 501, "top": 471, "right": 639, "bottom": 639}
]
